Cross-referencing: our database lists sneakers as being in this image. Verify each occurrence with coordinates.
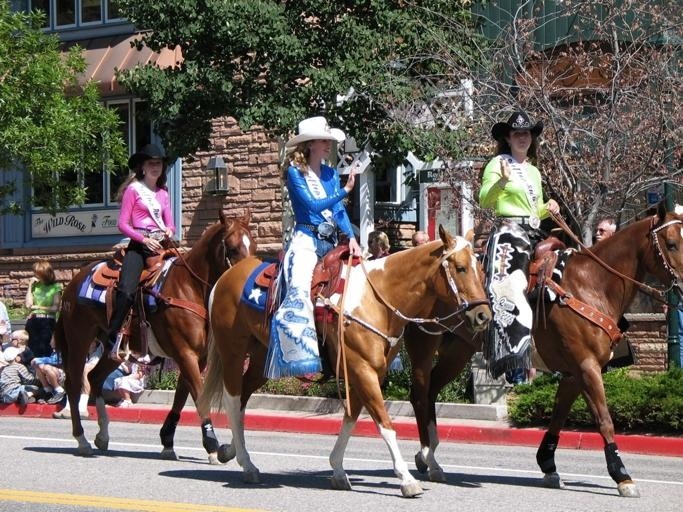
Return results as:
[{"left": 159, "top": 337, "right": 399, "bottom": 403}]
[
  {"left": 117, "top": 398, "right": 133, "bottom": 408},
  {"left": 54, "top": 408, "right": 88, "bottom": 419},
  {"left": 19, "top": 387, "right": 66, "bottom": 404}
]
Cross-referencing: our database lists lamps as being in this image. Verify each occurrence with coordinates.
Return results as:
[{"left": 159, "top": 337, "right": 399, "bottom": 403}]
[{"left": 202, "top": 157, "right": 231, "bottom": 197}]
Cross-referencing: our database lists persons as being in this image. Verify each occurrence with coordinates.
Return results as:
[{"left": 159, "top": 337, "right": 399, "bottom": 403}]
[
  {"left": 0, "top": 261, "right": 151, "bottom": 419},
  {"left": 368, "top": 231, "right": 391, "bottom": 260},
  {"left": 596, "top": 217, "right": 616, "bottom": 244},
  {"left": 261, "top": 116, "right": 362, "bottom": 379},
  {"left": 105, "top": 144, "right": 177, "bottom": 358},
  {"left": 478, "top": 111, "right": 560, "bottom": 387},
  {"left": 411, "top": 230, "right": 430, "bottom": 246}
]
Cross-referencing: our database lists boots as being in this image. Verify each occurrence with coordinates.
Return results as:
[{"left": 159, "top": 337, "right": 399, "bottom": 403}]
[{"left": 102, "top": 290, "right": 134, "bottom": 351}]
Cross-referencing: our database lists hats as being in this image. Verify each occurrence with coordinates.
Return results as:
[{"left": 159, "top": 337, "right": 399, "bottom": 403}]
[
  {"left": 4, "top": 347, "right": 25, "bottom": 361},
  {"left": 491, "top": 112, "right": 543, "bottom": 141},
  {"left": 128, "top": 144, "right": 177, "bottom": 170},
  {"left": 286, "top": 117, "right": 346, "bottom": 147}
]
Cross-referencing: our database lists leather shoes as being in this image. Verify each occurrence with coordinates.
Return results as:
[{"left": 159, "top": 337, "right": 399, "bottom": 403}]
[{"left": 506, "top": 367, "right": 524, "bottom": 383}]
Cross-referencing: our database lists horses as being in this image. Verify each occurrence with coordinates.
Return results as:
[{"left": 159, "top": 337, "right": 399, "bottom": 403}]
[
  {"left": 52, "top": 206, "right": 258, "bottom": 467},
  {"left": 192, "top": 224, "right": 493, "bottom": 496},
  {"left": 401, "top": 197, "right": 683, "bottom": 499}
]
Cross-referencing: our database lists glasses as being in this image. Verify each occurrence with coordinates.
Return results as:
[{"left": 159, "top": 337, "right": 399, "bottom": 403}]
[{"left": 595, "top": 227, "right": 605, "bottom": 234}]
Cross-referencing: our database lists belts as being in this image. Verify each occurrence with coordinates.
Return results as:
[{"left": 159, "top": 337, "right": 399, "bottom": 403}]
[
  {"left": 504, "top": 215, "right": 542, "bottom": 229},
  {"left": 300, "top": 222, "right": 337, "bottom": 236}
]
